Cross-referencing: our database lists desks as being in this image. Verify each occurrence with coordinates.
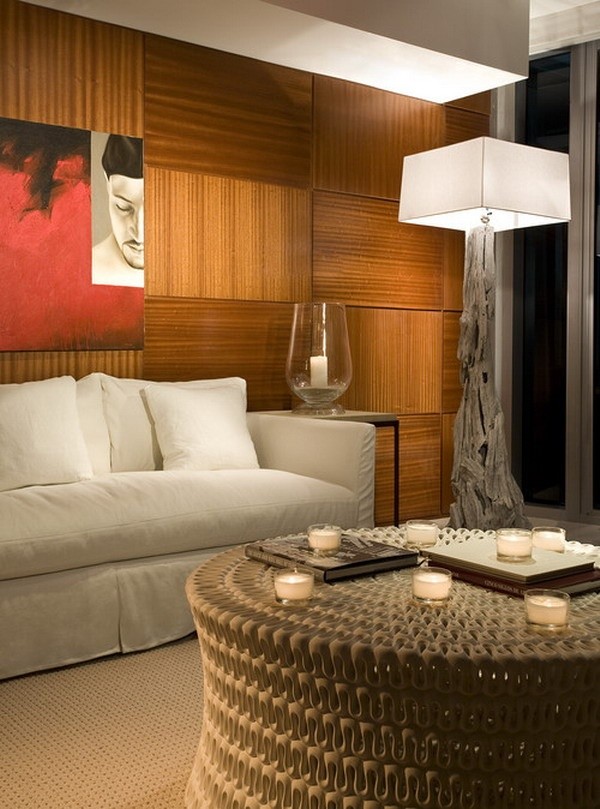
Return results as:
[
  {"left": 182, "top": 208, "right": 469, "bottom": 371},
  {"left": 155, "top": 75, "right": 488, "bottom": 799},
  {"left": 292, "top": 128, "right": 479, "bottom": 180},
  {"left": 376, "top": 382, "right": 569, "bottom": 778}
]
[{"left": 250, "top": 410, "right": 400, "bottom": 527}]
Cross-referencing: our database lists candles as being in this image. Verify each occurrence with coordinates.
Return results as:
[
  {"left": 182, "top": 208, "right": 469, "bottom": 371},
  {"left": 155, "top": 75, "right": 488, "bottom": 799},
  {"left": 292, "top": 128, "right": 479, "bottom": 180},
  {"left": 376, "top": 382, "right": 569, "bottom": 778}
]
[
  {"left": 408, "top": 525, "right": 435, "bottom": 544},
  {"left": 527, "top": 595, "right": 568, "bottom": 624},
  {"left": 311, "top": 529, "right": 339, "bottom": 549},
  {"left": 414, "top": 571, "right": 448, "bottom": 598},
  {"left": 310, "top": 356, "right": 328, "bottom": 388},
  {"left": 535, "top": 531, "right": 564, "bottom": 550},
  {"left": 276, "top": 570, "right": 312, "bottom": 600},
  {"left": 498, "top": 534, "right": 533, "bottom": 558}
]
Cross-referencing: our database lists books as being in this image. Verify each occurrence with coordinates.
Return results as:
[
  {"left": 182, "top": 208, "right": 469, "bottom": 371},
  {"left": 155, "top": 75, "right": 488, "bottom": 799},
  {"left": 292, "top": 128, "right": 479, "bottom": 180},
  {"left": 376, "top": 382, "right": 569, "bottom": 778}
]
[
  {"left": 246, "top": 533, "right": 419, "bottom": 582},
  {"left": 420, "top": 538, "right": 595, "bottom": 583},
  {"left": 428, "top": 559, "right": 600, "bottom": 598}
]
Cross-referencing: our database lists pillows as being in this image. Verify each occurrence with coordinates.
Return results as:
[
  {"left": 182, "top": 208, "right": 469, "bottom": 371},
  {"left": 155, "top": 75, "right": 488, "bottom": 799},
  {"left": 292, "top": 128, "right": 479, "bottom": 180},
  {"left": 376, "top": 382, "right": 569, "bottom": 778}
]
[{"left": 0, "top": 372, "right": 262, "bottom": 492}]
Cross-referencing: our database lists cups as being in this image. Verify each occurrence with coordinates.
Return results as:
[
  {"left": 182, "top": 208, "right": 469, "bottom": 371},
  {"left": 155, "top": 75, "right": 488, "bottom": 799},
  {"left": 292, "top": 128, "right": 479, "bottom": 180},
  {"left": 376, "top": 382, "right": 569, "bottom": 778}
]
[
  {"left": 412, "top": 566, "right": 452, "bottom": 605},
  {"left": 532, "top": 527, "right": 566, "bottom": 554},
  {"left": 306, "top": 523, "right": 342, "bottom": 554},
  {"left": 406, "top": 520, "right": 438, "bottom": 549},
  {"left": 524, "top": 589, "right": 571, "bottom": 631},
  {"left": 285, "top": 300, "right": 354, "bottom": 416},
  {"left": 496, "top": 527, "right": 533, "bottom": 562},
  {"left": 273, "top": 568, "right": 315, "bottom": 606}
]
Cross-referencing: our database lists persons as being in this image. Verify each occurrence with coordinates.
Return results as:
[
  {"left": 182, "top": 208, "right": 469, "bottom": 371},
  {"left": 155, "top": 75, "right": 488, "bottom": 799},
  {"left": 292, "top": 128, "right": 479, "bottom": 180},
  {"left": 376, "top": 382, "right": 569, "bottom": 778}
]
[{"left": 91, "top": 131, "right": 143, "bottom": 287}]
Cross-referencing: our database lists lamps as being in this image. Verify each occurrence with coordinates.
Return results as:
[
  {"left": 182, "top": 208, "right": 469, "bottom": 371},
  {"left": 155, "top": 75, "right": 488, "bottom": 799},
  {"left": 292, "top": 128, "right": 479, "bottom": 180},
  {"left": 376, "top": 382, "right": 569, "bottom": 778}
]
[{"left": 398, "top": 136, "right": 574, "bottom": 525}]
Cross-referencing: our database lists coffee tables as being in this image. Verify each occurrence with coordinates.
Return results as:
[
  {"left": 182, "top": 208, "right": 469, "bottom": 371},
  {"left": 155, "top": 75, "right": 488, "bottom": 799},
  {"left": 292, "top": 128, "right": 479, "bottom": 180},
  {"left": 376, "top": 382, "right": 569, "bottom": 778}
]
[{"left": 185, "top": 525, "right": 600, "bottom": 809}]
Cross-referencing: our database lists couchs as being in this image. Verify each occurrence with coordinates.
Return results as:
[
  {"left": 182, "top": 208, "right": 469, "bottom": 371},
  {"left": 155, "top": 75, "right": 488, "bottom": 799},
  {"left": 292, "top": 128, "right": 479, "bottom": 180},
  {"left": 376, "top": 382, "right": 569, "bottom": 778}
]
[{"left": 0, "top": 412, "right": 378, "bottom": 682}]
